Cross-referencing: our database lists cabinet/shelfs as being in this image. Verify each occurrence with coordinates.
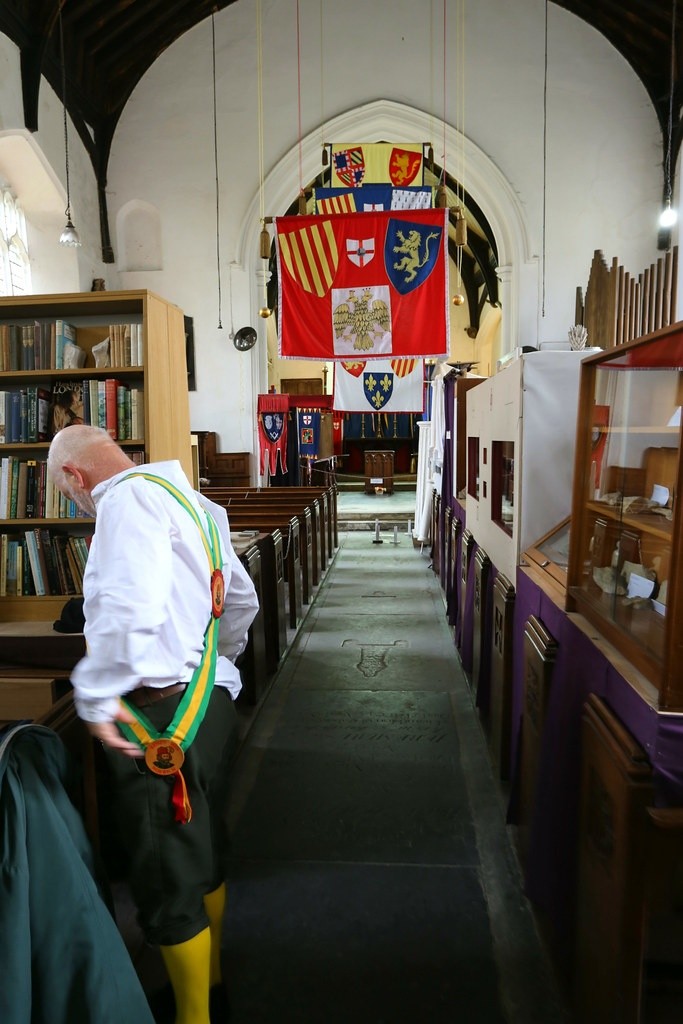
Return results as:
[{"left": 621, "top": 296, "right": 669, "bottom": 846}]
[
  {"left": 0, "top": 289, "right": 194, "bottom": 722},
  {"left": 563, "top": 313, "right": 683, "bottom": 713}
]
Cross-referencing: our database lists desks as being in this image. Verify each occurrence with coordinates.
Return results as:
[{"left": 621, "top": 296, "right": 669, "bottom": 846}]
[{"left": 365, "top": 451, "right": 396, "bottom": 496}]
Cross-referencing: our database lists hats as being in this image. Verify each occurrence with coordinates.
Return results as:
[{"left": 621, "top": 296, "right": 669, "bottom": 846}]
[{"left": 52, "top": 597, "right": 85, "bottom": 633}]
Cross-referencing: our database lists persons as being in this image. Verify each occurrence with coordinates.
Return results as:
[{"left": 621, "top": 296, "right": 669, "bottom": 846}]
[{"left": 48, "top": 424, "right": 260, "bottom": 1024}]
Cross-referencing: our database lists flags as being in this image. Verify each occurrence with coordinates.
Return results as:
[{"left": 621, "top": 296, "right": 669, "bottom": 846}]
[
  {"left": 297, "top": 408, "right": 321, "bottom": 460},
  {"left": 272, "top": 141, "right": 451, "bottom": 414}
]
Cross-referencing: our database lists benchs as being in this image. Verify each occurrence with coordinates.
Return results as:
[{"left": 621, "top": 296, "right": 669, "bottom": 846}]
[{"left": 198, "top": 484, "right": 339, "bottom": 710}]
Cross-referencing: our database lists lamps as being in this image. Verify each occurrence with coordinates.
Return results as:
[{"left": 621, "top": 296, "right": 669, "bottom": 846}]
[{"left": 58, "top": 5, "right": 82, "bottom": 248}]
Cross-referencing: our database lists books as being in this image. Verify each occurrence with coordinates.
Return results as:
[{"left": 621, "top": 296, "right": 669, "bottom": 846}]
[{"left": 0, "top": 320, "right": 144, "bottom": 597}]
